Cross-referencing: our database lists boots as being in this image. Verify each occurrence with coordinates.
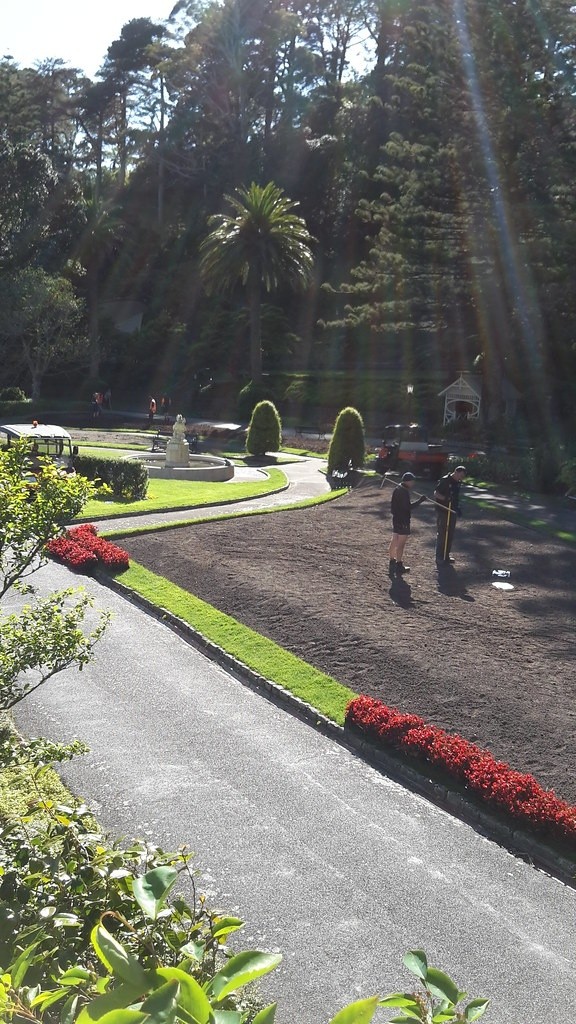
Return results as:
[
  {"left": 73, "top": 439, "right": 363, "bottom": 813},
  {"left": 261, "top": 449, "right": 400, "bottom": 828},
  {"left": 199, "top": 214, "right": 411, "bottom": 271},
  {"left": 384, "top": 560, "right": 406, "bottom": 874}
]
[
  {"left": 389, "top": 559, "right": 396, "bottom": 572},
  {"left": 395, "top": 562, "right": 410, "bottom": 573}
]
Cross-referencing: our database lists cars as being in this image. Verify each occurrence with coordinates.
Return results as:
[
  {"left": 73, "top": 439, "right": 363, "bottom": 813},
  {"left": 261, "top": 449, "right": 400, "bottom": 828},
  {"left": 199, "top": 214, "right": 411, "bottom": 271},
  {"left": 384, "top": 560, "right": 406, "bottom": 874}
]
[{"left": 0, "top": 421, "right": 79, "bottom": 489}]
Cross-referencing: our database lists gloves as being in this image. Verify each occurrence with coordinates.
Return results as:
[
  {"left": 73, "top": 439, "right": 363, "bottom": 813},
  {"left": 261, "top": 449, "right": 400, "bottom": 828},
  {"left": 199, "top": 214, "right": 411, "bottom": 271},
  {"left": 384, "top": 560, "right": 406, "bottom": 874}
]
[
  {"left": 445, "top": 496, "right": 453, "bottom": 502},
  {"left": 418, "top": 494, "right": 429, "bottom": 504},
  {"left": 457, "top": 509, "right": 462, "bottom": 519}
]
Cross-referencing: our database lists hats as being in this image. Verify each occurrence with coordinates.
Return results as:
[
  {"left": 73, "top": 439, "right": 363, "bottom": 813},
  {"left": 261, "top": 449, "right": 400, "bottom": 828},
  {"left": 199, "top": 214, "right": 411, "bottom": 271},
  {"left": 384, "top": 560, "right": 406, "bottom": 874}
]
[{"left": 402, "top": 472, "right": 416, "bottom": 481}]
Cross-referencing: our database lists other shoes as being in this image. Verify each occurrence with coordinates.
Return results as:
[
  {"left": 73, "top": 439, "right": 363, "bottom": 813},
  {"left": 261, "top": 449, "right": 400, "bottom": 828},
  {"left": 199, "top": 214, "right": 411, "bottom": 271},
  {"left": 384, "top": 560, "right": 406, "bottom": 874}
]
[{"left": 434, "top": 556, "right": 454, "bottom": 565}]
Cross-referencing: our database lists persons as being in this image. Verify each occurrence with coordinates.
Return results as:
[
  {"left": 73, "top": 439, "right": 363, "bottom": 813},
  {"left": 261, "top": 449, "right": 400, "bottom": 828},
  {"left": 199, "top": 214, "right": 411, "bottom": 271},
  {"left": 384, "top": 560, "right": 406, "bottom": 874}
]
[
  {"left": 92, "top": 392, "right": 105, "bottom": 417},
  {"left": 388, "top": 472, "right": 426, "bottom": 572},
  {"left": 434, "top": 466, "right": 466, "bottom": 563},
  {"left": 148, "top": 396, "right": 157, "bottom": 424},
  {"left": 161, "top": 393, "right": 171, "bottom": 421}
]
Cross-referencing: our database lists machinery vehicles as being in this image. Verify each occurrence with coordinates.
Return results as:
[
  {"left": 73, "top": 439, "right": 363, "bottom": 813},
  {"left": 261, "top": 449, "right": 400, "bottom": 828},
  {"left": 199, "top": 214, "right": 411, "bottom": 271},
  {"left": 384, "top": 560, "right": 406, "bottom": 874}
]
[{"left": 370, "top": 423, "right": 449, "bottom": 480}]
